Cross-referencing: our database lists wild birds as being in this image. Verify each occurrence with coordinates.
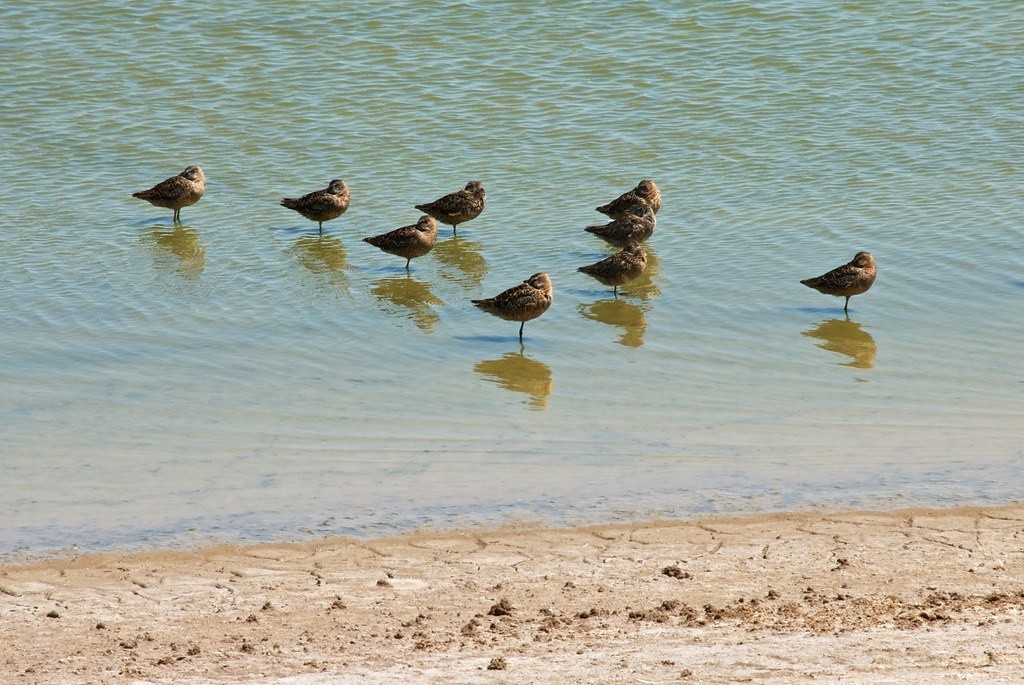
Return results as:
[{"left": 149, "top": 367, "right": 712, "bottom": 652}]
[
  {"left": 361, "top": 216, "right": 438, "bottom": 266},
  {"left": 574, "top": 240, "right": 647, "bottom": 293},
  {"left": 415, "top": 179, "right": 486, "bottom": 228},
  {"left": 594, "top": 179, "right": 661, "bottom": 218},
  {"left": 279, "top": 178, "right": 350, "bottom": 228},
  {"left": 469, "top": 272, "right": 552, "bottom": 332},
  {"left": 583, "top": 196, "right": 656, "bottom": 248},
  {"left": 131, "top": 164, "right": 206, "bottom": 220},
  {"left": 800, "top": 251, "right": 877, "bottom": 309}
]
